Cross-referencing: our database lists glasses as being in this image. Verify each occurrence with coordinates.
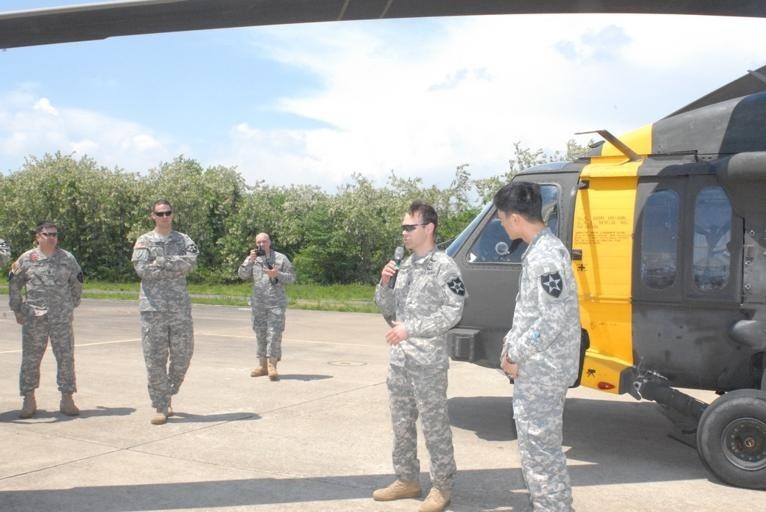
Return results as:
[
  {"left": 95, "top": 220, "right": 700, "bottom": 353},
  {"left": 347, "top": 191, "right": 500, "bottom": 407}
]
[
  {"left": 154, "top": 211, "right": 172, "bottom": 217},
  {"left": 39, "top": 232, "right": 56, "bottom": 237},
  {"left": 400, "top": 223, "right": 426, "bottom": 232}
]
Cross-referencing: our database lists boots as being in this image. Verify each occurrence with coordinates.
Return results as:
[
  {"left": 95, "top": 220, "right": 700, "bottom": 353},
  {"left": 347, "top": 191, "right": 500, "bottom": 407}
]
[
  {"left": 250, "top": 356, "right": 281, "bottom": 380},
  {"left": 59, "top": 392, "right": 80, "bottom": 416},
  {"left": 151, "top": 395, "right": 174, "bottom": 425},
  {"left": 374, "top": 476, "right": 420, "bottom": 500},
  {"left": 418, "top": 484, "right": 453, "bottom": 512},
  {"left": 21, "top": 395, "right": 37, "bottom": 418}
]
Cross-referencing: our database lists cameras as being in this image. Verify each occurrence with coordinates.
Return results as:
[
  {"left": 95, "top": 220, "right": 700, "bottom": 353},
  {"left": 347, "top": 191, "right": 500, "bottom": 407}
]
[{"left": 255, "top": 246, "right": 266, "bottom": 256}]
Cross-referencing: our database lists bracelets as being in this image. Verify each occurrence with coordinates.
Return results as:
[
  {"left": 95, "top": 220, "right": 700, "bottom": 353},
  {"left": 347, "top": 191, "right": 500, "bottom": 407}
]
[{"left": 506, "top": 352, "right": 514, "bottom": 364}]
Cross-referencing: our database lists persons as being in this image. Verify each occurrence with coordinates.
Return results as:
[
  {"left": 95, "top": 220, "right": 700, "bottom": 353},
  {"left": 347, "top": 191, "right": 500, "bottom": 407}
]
[
  {"left": 132, "top": 199, "right": 201, "bottom": 424},
  {"left": 373, "top": 199, "right": 468, "bottom": 511},
  {"left": 237, "top": 234, "right": 294, "bottom": 381},
  {"left": 493, "top": 181, "right": 581, "bottom": 512},
  {"left": 8, "top": 222, "right": 82, "bottom": 418}
]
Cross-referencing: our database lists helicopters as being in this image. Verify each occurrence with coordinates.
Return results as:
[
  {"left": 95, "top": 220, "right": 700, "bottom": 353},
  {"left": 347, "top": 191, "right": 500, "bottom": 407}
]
[{"left": 0, "top": 2, "right": 766, "bottom": 491}]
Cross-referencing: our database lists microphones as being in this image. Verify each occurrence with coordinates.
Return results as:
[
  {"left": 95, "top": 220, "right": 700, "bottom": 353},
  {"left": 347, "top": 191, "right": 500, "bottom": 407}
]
[{"left": 387, "top": 246, "right": 404, "bottom": 289}]
